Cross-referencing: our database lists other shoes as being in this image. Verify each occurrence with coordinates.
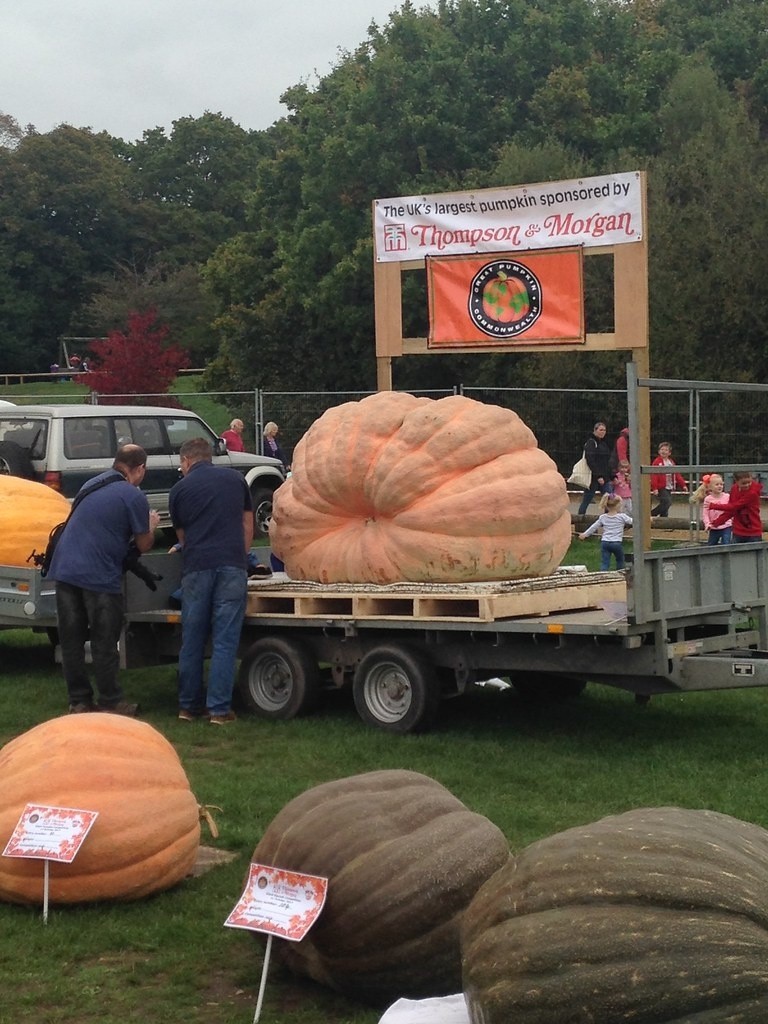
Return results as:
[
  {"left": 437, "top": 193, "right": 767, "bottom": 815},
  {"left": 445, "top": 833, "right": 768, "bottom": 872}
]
[
  {"left": 68, "top": 702, "right": 96, "bottom": 712},
  {"left": 250, "top": 566, "right": 273, "bottom": 578},
  {"left": 98, "top": 702, "right": 142, "bottom": 715},
  {"left": 211, "top": 709, "right": 237, "bottom": 724},
  {"left": 178, "top": 708, "right": 208, "bottom": 722}
]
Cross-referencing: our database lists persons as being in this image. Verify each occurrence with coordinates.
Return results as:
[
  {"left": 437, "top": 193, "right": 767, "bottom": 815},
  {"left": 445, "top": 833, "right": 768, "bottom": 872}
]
[
  {"left": 222, "top": 419, "right": 246, "bottom": 451},
  {"left": 167, "top": 438, "right": 253, "bottom": 723},
  {"left": 50, "top": 444, "right": 156, "bottom": 719},
  {"left": 261, "top": 422, "right": 290, "bottom": 477},
  {"left": 579, "top": 422, "right": 763, "bottom": 574}
]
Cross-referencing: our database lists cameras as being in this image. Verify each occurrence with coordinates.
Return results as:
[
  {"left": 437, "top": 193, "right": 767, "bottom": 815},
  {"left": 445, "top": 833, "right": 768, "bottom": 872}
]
[{"left": 122, "top": 543, "right": 163, "bottom": 593}]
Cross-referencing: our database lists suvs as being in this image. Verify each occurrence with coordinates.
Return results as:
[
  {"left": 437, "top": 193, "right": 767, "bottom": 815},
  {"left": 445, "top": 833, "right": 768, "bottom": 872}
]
[{"left": 0, "top": 401, "right": 290, "bottom": 546}]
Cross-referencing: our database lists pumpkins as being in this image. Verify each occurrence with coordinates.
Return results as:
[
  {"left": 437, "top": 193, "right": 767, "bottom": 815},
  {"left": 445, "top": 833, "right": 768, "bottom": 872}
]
[
  {"left": 241, "top": 769, "right": 514, "bottom": 1002},
  {"left": 266, "top": 391, "right": 572, "bottom": 586},
  {"left": 0, "top": 710, "right": 218, "bottom": 906},
  {"left": 0, "top": 474, "right": 73, "bottom": 589},
  {"left": 460, "top": 807, "right": 768, "bottom": 1024}
]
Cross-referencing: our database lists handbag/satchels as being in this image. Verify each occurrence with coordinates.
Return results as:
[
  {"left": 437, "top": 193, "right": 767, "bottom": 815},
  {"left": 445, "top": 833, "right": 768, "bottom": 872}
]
[{"left": 567, "top": 458, "right": 592, "bottom": 490}]
